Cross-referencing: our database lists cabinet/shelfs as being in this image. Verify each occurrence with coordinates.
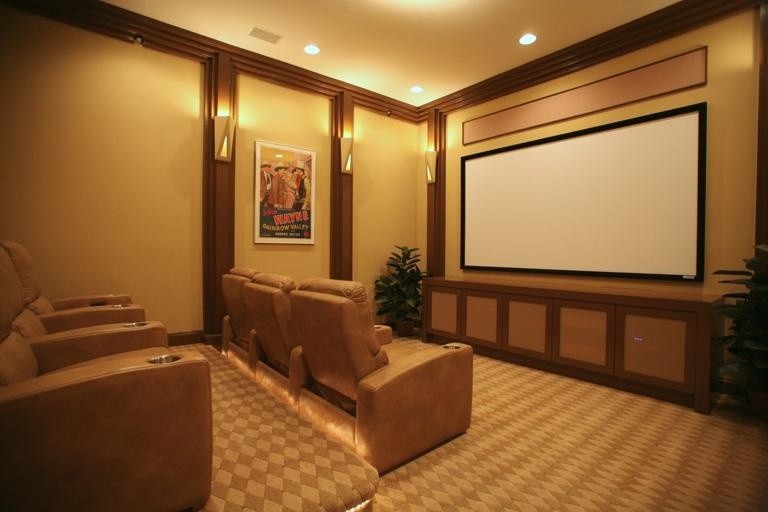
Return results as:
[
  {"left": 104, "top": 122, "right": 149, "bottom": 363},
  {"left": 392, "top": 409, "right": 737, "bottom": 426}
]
[{"left": 422, "top": 275, "right": 724, "bottom": 415}]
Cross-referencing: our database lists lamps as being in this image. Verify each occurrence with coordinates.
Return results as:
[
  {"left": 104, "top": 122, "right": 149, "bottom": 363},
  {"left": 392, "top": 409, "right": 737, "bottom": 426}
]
[
  {"left": 213, "top": 116, "right": 234, "bottom": 164},
  {"left": 425, "top": 150, "right": 436, "bottom": 183},
  {"left": 341, "top": 138, "right": 354, "bottom": 174}
]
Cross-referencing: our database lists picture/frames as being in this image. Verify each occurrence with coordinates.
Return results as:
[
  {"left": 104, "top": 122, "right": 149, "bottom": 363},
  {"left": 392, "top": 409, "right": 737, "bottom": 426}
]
[{"left": 253, "top": 140, "right": 316, "bottom": 245}]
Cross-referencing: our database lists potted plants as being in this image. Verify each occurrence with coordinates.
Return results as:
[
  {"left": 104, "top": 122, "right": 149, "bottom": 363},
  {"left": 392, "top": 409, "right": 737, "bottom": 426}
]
[
  {"left": 708, "top": 243, "right": 768, "bottom": 421},
  {"left": 372, "top": 244, "right": 432, "bottom": 338}
]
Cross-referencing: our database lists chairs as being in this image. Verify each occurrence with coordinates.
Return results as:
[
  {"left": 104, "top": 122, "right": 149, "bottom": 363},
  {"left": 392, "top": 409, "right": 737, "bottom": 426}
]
[
  {"left": 1, "top": 240, "right": 144, "bottom": 314},
  {"left": 289, "top": 279, "right": 474, "bottom": 480},
  {"left": 0, "top": 261, "right": 212, "bottom": 511},
  {"left": 242, "top": 273, "right": 294, "bottom": 390},
  {"left": 220, "top": 267, "right": 257, "bottom": 374},
  {"left": 0, "top": 247, "right": 167, "bottom": 339}
]
